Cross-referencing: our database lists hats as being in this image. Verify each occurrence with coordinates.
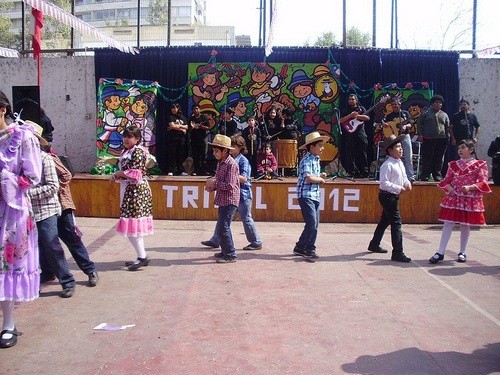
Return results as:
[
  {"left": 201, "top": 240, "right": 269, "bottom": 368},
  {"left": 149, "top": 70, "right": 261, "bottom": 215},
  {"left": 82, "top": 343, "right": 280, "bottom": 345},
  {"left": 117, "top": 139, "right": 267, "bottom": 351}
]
[
  {"left": 207, "top": 134, "right": 235, "bottom": 150},
  {"left": 23, "top": 120, "right": 48, "bottom": 146},
  {"left": 380, "top": 134, "right": 405, "bottom": 157},
  {"left": 299, "top": 132, "right": 331, "bottom": 150}
]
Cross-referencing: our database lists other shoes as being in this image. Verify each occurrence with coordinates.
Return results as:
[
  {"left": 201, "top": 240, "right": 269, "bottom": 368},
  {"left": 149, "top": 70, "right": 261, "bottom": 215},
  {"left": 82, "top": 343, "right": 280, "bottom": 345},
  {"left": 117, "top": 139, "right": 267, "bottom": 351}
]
[
  {"left": 62, "top": 282, "right": 74, "bottom": 297},
  {"left": 88, "top": 270, "right": 98, "bottom": 285},
  {"left": 214, "top": 253, "right": 224, "bottom": 257},
  {"left": 243, "top": 243, "right": 262, "bottom": 250},
  {"left": 125, "top": 261, "right": 149, "bottom": 266},
  {"left": 129, "top": 257, "right": 148, "bottom": 270},
  {"left": 200, "top": 241, "right": 219, "bottom": 248},
  {"left": 368, "top": 245, "right": 388, "bottom": 253},
  {"left": 216, "top": 257, "right": 236, "bottom": 262},
  {"left": 391, "top": 253, "right": 411, "bottom": 262}
]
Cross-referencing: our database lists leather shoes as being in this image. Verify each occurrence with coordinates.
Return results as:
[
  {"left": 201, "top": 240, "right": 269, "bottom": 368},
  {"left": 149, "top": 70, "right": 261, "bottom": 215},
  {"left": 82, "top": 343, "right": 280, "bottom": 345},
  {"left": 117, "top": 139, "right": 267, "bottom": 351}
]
[
  {"left": 430, "top": 252, "right": 444, "bottom": 263},
  {"left": 0, "top": 328, "right": 23, "bottom": 348},
  {"left": 457, "top": 253, "right": 466, "bottom": 261}
]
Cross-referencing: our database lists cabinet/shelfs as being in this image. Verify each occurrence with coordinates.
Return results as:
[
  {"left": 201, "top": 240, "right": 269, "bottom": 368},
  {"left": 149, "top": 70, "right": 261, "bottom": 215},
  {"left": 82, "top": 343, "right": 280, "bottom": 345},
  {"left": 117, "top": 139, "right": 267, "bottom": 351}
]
[{"left": 374, "top": 141, "right": 420, "bottom": 179}]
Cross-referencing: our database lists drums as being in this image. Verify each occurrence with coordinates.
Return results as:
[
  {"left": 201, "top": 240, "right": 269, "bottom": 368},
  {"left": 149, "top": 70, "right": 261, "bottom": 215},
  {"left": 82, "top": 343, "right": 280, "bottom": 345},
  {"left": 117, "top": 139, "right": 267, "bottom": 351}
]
[
  {"left": 271, "top": 139, "right": 298, "bottom": 169},
  {"left": 395, "top": 117, "right": 415, "bottom": 140}
]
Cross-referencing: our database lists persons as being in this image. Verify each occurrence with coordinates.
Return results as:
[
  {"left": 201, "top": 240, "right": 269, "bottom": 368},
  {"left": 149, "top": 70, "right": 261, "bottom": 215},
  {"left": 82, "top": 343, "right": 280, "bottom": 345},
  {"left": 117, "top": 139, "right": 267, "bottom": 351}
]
[
  {"left": 452, "top": 98, "right": 480, "bottom": 161},
  {"left": 110, "top": 125, "right": 154, "bottom": 271},
  {"left": 189, "top": 105, "right": 211, "bottom": 176},
  {"left": 0, "top": 90, "right": 99, "bottom": 347},
  {"left": 416, "top": 95, "right": 450, "bottom": 180},
  {"left": 202, "top": 136, "right": 262, "bottom": 250},
  {"left": 242, "top": 117, "right": 261, "bottom": 179},
  {"left": 263, "top": 105, "right": 284, "bottom": 177},
  {"left": 367, "top": 134, "right": 413, "bottom": 262},
  {"left": 204, "top": 134, "right": 241, "bottom": 262},
  {"left": 382, "top": 97, "right": 415, "bottom": 182},
  {"left": 429, "top": 141, "right": 491, "bottom": 263},
  {"left": 340, "top": 93, "right": 370, "bottom": 177},
  {"left": 219, "top": 107, "right": 237, "bottom": 136},
  {"left": 164, "top": 103, "right": 188, "bottom": 176},
  {"left": 293, "top": 132, "right": 330, "bottom": 259},
  {"left": 255, "top": 143, "right": 281, "bottom": 179}
]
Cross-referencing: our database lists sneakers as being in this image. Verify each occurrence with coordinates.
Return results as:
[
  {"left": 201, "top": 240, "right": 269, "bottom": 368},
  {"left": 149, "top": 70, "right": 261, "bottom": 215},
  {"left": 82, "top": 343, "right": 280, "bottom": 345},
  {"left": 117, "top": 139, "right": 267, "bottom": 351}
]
[
  {"left": 292, "top": 246, "right": 305, "bottom": 254},
  {"left": 303, "top": 249, "right": 316, "bottom": 257}
]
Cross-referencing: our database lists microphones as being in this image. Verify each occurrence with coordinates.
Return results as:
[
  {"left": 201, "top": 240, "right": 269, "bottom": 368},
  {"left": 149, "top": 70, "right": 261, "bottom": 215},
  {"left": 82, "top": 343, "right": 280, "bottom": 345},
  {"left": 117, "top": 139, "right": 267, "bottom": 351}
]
[{"left": 333, "top": 107, "right": 339, "bottom": 111}]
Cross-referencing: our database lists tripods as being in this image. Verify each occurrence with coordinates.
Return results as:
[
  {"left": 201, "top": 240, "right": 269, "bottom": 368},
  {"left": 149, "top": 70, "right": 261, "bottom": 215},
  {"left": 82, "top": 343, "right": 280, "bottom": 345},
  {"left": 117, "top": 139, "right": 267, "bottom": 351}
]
[
  {"left": 329, "top": 111, "right": 355, "bottom": 182},
  {"left": 252, "top": 139, "right": 283, "bottom": 181}
]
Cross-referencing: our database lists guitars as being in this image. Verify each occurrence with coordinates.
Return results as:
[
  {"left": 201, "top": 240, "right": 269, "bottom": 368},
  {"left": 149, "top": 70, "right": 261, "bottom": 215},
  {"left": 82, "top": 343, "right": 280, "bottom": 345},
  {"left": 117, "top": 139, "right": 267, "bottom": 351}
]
[
  {"left": 369, "top": 109, "right": 425, "bottom": 149},
  {"left": 342, "top": 94, "right": 392, "bottom": 135}
]
